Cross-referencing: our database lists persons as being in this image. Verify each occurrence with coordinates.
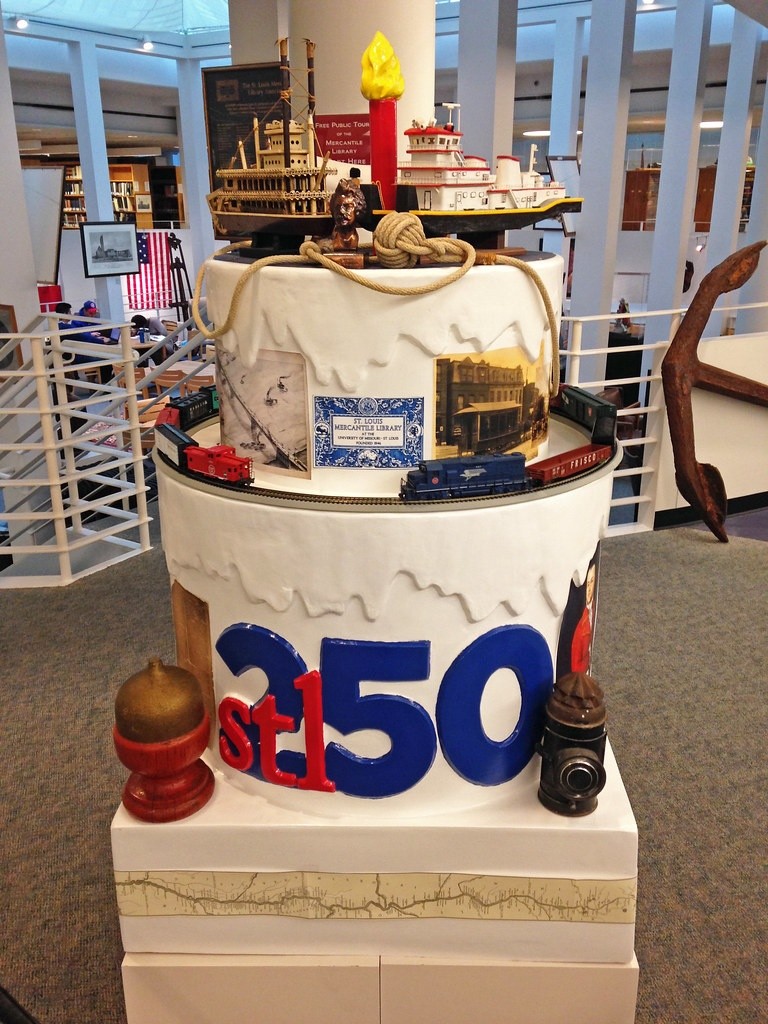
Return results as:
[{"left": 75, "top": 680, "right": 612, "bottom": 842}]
[
  {"left": 52, "top": 300, "right": 173, "bottom": 460},
  {"left": 329, "top": 177, "right": 367, "bottom": 251}
]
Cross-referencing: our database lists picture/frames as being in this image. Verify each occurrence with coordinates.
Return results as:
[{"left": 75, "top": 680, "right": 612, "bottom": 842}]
[
  {"left": 135, "top": 194, "right": 153, "bottom": 214},
  {"left": 78, "top": 221, "right": 141, "bottom": 278}
]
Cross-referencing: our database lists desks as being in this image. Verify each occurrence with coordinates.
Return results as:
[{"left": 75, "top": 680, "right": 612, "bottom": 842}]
[
  {"left": 99, "top": 336, "right": 166, "bottom": 362},
  {"left": 144, "top": 359, "right": 216, "bottom": 387}
]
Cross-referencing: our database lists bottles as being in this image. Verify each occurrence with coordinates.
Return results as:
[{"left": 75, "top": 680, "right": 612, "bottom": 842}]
[{"left": 139, "top": 328, "right": 150, "bottom": 344}]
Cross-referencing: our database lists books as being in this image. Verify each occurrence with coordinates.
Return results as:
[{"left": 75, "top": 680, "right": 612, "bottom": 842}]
[
  {"left": 738, "top": 171, "right": 754, "bottom": 232},
  {"left": 62, "top": 166, "right": 135, "bottom": 225}
]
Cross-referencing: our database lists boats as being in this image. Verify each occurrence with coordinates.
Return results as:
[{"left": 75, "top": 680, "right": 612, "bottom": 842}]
[
  {"left": 206, "top": 35, "right": 338, "bottom": 237},
  {"left": 372, "top": 102, "right": 581, "bottom": 234}
]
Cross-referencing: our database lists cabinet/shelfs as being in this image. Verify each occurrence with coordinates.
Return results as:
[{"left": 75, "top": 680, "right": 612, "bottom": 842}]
[
  {"left": 621, "top": 168, "right": 661, "bottom": 231},
  {"left": 148, "top": 166, "right": 186, "bottom": 229},
  {"left": 694, "top": 167, "right": 755, "bottom": 232},
  {"left": 62, "top": 163, "right": 154, "bottom": 229}
]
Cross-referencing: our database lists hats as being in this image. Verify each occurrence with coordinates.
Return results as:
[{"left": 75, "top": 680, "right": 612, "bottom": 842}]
[{"left": 84, "top": 300, "right": 96, "bottom": 308}]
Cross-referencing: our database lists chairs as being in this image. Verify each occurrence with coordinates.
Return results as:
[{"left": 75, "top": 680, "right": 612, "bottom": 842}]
[
  {"left": 77, "top": 367, "right": 102, "bottom": 384},
  {"left": 616, "top": 419, "right": 642, "bottom": 448},
  {"left": 124, "top": 394, "right": 170, "bottom": 424},
  {"left": 122, "top": 426, "right": 155, "bottom": 449},
  {"left": 163, "top": 321, "right": 179, "bottom": 341},
  {"left": 150, "top": 366, "right": 185, "bottom": 397},
  {"left": 184, "top": 373, "right": 215, "bottom": 394},
  {"left": 205, "top": 344, "right": 216, "bottom": 362},
  {"left": 112, "top": 364, "right": 149, "bottom": 400}
]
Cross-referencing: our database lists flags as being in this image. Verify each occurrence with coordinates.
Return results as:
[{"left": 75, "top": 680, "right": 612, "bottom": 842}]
[{"left": 127, "top": 231, "right": 172, "bottom": 310}]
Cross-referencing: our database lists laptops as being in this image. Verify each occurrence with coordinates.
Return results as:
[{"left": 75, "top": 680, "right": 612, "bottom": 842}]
[{"left": 105, "top": 328, "right": 121, "bottom": 345}]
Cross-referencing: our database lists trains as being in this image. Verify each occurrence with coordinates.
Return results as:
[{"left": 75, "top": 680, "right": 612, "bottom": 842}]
[
  {"left": 399, "top": 382, "right": 618, "bottom": 502},
  {"left": 153, "top": 384, "right": 254, "bottom": 488}
]
